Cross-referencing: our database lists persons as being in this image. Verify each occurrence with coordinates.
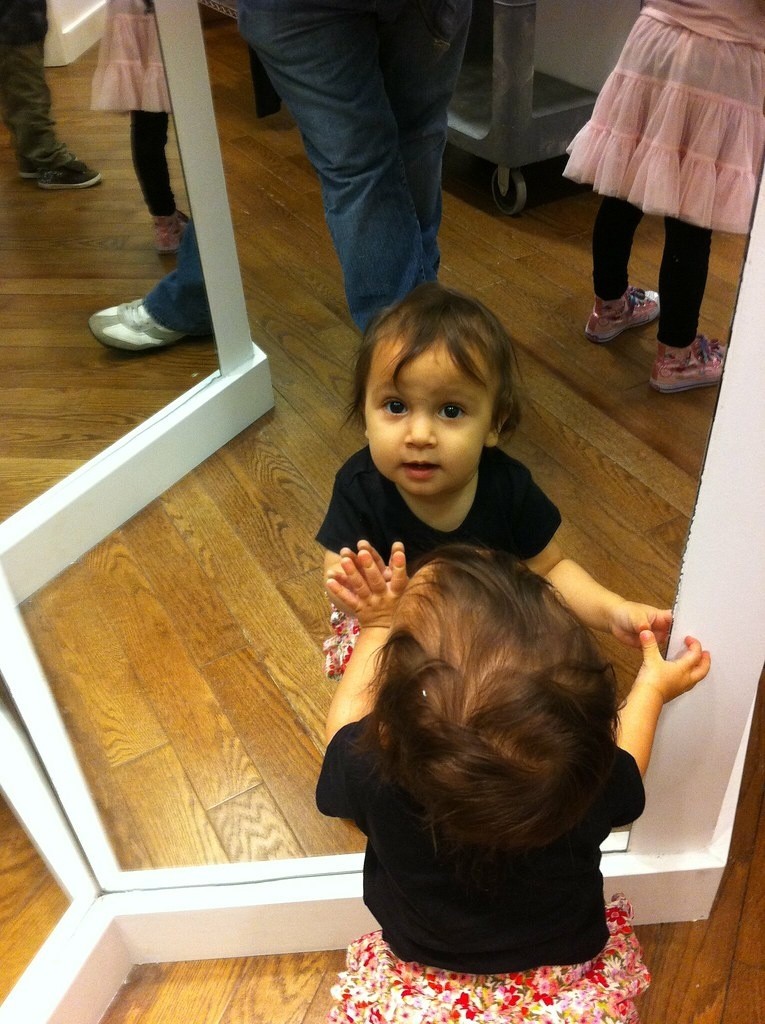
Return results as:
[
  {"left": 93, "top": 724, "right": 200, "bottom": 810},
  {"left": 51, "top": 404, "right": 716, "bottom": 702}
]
[
  {"left": 557, "top": 0, "right": 764, "bottom": 393},
  {"left": 89, "top": 0, "right": 189, "bottom": 254},
  {"left": 236, "top": 0, "right": 473, "bottom": 334},
  {"left": 88, "top": 215, "right": 215, "bottom": 352},
  {"left": 321, "top": 289, "right": 674, "bottom": 681},
  {"left": 0, "top": 0, "right": 102, "bottom": 190},
  {"left": 313, "top": 548, "right": 711, "bottom": 1024}
]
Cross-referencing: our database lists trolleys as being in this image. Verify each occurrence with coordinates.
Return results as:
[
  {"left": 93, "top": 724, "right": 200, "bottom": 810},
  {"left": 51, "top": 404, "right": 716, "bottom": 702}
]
[{"left": 451, "top": 1, "right": 643, "bottom": 227}]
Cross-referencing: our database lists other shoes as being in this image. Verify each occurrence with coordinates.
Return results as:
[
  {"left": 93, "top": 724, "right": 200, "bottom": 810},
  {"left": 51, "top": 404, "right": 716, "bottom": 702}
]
[
  {"left": 17, "top": 149, "right": 77, "bottom": 178},
  {"left": 38, "top": 160, "right": 101, "bottom": 188},
  {"left": 154, "top": 210, "right": 190, "bottom": 254}
]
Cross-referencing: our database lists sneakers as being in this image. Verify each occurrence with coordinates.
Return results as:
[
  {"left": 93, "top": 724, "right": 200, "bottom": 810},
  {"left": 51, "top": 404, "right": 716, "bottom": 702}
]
[
  {"left": 650, "top": 334, "right": 727, "bottom": 393},
  {"left": 86, "top": 299, "right": 214, "bottom": 352},
  {"left": 585, "top": 286, "right": 660, "bottom": 342}
]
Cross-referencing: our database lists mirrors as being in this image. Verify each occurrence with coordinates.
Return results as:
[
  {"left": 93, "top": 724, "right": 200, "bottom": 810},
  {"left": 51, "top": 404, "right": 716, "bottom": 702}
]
[
  {"left": 0, "top": 0, "right": 765, "bottom": 892},
  {"left": 0, "top": 0, "right": 274, "bottom": 608}
]
